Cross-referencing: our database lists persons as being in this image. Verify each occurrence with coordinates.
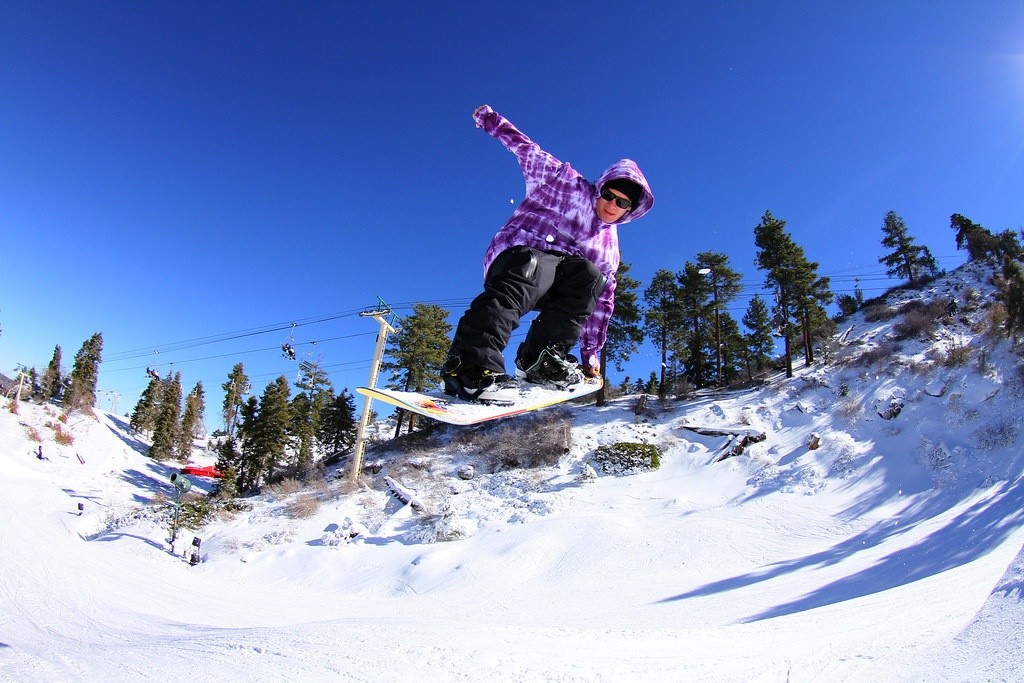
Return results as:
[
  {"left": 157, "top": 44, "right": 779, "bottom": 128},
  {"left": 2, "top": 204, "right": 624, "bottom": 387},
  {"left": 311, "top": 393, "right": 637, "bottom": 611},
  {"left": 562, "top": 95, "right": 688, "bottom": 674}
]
[{"left": 439, "top": 104, "right": 654, "bottom": 407}]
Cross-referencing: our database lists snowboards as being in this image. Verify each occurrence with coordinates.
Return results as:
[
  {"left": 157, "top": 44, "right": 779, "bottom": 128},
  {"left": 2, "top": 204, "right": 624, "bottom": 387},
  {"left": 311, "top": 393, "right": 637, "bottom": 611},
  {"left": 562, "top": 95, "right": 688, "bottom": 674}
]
[{"left": 356, "top": 368, "right": 606, "bottom": 427}]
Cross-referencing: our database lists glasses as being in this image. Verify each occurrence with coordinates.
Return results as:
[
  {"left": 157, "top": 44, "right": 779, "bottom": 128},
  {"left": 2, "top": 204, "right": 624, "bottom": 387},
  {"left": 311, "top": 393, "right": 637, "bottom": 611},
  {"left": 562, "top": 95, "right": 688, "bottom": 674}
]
[{"left": 601, "top": 187, "right": 633, "bottom": 209}]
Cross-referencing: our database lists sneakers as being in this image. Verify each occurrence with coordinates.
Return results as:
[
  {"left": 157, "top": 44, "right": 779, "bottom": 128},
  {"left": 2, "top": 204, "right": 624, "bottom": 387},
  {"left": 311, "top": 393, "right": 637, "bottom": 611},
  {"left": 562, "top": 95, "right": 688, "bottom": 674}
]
[
  {"left": 440, "top": 354, "right": 520, "bottom": 406},
  {"left": 514, "top": 346, "right": 585, "bottom": 391}
]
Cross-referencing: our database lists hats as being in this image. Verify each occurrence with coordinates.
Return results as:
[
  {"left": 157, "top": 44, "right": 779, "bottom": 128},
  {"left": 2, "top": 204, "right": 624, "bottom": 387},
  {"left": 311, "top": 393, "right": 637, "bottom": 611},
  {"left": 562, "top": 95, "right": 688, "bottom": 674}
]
[{"left": 601, "top": 179, "right": 643, "bottom": 207}]
